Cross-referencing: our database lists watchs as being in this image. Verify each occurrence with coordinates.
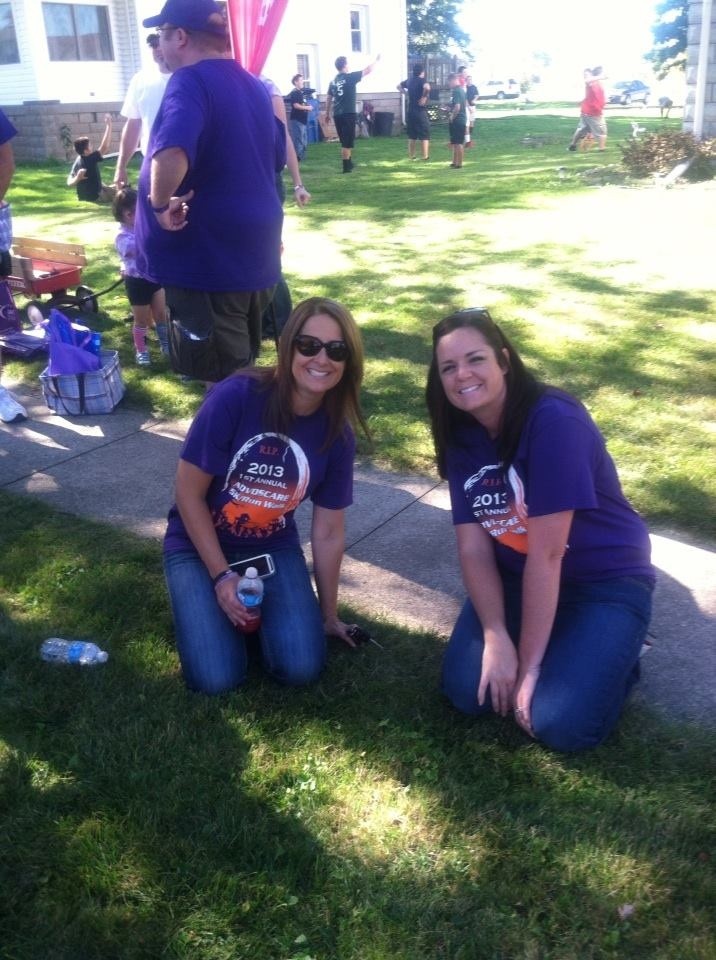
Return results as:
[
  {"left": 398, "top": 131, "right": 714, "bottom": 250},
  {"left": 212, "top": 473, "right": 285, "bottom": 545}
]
[{"left": 147, "top": 194, "right": 170, "bottom": 213}]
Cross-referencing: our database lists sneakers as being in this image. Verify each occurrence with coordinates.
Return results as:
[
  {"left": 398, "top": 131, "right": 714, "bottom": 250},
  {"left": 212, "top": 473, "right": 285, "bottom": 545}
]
[
  {"left": 135, "top": 351, "right": 153, "bottom": 366},
  {"left": 0, "top": 384, "right": 28, "bottom": 424}
]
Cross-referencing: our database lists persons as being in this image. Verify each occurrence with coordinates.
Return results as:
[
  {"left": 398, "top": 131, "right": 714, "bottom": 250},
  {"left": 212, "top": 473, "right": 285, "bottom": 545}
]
[
  {"left": 0, "top": 107, "right": 29, "bottom": 424},
  {"left": 162, "top": 298, "right": 361, "bottom": 695},
  {"left": 659, "top": 97, "right": 673, "bottom": 117},
  {"left": 138, "top": 0, "right": 287, "bottom": 383},
  {"left": 569, "top": 66, "right": 612, "bottom": 153},
  {"left": 426, "top": 311, "right": 657, "bottom": 755},
  {"left": 112, "top": 188, "right": 171, "bottom": 366},
  {"left": 65, "top": 33, "right": 311, "bottom": 255},
  {"left": 289, "top": 52, "right": 480, "bottom": 173}
]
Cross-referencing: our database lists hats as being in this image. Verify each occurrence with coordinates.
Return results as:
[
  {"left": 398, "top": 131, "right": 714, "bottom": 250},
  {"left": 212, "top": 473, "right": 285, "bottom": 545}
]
[{"left": 142, "top": 0, "right": 226, "bottom": 36}]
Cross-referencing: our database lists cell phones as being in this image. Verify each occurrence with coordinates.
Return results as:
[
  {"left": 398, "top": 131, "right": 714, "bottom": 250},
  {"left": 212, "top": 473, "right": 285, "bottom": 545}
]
[{"left": 228, "top": 554, "right": 276, "bottom": 579}]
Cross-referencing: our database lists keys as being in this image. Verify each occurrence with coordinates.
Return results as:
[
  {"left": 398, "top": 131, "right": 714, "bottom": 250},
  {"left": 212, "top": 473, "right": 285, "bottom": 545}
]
[{"left": 349, "top": 625, "right": 384, "bottom": 650}]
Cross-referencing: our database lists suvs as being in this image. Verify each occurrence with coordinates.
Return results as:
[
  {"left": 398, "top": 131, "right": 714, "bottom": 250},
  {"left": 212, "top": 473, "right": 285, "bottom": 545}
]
[{"left": 477, "top": 76, "right": 521, "bottom": 100}]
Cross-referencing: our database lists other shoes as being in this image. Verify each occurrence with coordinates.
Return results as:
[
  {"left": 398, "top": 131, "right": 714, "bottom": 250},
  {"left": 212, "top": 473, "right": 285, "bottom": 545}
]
[
  {"left": 410, "top": 157, "right": 430, "bottom": 161},
  {"left": 465, "top": 141, "right": 472, "bottom": 149},
  {"left": 566, "top": 144, "right": 577, "bottom": 152},
  {"left": 449, "top": 163, "right": 462, "bottom": 169},
  {"left": 343, "top": 156, "right": 353, "bottom": 172}
]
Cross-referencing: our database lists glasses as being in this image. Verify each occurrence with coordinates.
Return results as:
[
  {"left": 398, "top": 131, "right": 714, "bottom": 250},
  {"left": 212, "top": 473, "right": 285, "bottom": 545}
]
[
  {"left": 156, "top": 25, "right": 191, "bottom": 36},
  {"left": 455, "top": 307, "right": 492, "bottom": 322},
  {"left": 295, "top": 334, "right": 349, "bottom": 362}
]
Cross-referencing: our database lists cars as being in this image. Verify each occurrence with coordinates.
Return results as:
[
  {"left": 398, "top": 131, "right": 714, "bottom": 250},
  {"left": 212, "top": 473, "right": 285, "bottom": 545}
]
[{"left": 605, "top": 78, "right": 652, "bottom": 106}]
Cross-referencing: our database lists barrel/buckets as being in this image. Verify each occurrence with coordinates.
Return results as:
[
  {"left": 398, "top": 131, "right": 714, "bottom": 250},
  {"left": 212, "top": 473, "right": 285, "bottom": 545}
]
[{"left": 368, "top": 111, "right": 395, "bottom": 137}]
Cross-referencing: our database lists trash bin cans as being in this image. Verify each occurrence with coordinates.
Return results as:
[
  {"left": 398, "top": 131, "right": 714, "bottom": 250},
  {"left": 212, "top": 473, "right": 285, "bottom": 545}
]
[{"left": 374, "top": 112, "right": 395, "bottom": 136}]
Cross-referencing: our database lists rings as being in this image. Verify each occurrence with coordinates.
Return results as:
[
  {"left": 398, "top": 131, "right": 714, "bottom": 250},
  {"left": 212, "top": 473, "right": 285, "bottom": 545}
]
[{"left": 513, "top": 706, "right": 524, "bottom": 713}]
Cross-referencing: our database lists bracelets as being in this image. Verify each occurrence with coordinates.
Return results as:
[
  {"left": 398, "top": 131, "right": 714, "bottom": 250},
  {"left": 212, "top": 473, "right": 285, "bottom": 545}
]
[
  {"left": 292, "top": 185, "right": 304, "bottom": 192},
  {"left": 213, "top": 569, "right": 233, "bottom": 584}
]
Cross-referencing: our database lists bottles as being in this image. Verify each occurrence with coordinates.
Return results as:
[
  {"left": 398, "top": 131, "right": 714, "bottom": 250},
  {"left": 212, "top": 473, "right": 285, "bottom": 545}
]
[
  {"left": 37, "top": 637, "right": 109, "bottom": 666},
  {"left": 236, "top": 567, "right": 264, "bottom": 634}
]
